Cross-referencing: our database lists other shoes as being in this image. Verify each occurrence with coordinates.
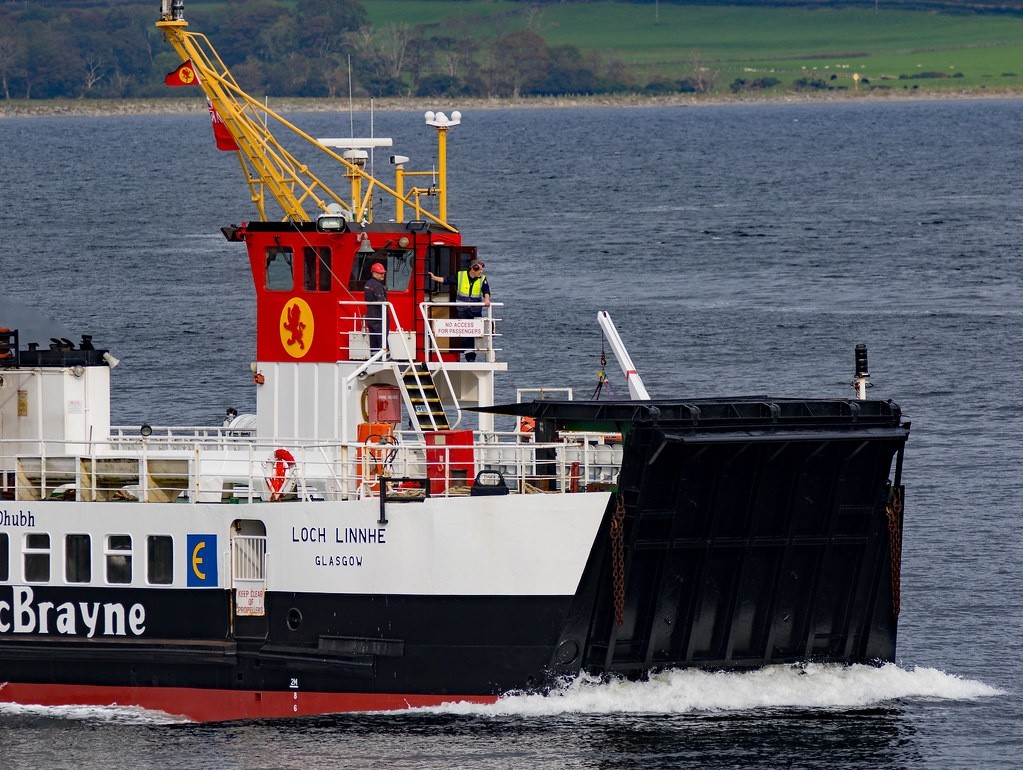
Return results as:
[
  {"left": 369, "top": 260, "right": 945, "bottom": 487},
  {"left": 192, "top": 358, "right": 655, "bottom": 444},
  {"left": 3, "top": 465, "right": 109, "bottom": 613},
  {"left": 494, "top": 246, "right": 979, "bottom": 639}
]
[{"left": 468, "top": 359, "right": 473, "bottom": 362}]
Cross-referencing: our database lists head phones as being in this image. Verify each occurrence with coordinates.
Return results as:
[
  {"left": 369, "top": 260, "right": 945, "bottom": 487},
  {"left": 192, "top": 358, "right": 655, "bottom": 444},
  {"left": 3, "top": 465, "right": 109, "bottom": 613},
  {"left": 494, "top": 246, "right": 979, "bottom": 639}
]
[{"left": 473, "top": 264, "right": 479, "bottom": 271}]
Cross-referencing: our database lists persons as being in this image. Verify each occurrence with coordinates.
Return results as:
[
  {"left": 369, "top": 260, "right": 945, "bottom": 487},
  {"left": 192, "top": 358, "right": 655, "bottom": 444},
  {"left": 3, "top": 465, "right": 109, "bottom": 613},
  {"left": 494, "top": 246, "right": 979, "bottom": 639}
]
[
  {"left": 514, "top": 416, "right": 536, "bottom": 443},
  {"left": 364, "top": 263, "right": 391, "bottom": 361},
  {"left": 221, "top": 408, "right": 238, "bottom": 436},
  {"left": 428, "top": 260, "right": 491, "bottom": 362}
]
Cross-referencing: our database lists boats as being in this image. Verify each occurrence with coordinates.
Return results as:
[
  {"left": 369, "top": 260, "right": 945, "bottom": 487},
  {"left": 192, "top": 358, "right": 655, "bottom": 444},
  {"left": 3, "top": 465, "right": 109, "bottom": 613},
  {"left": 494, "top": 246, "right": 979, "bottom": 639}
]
[{"left": 0, "top": 0, "right": 914, "bottom": 727}]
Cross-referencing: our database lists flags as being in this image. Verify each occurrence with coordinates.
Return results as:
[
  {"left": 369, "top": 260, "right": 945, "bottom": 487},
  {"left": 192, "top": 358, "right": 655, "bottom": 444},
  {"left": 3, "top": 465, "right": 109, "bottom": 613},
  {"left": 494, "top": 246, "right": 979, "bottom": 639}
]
[
  {"left": 163, "top": 59, "right": 202, "bottom": 86},
  {"left": 207, "top": 100, "right": 240, "bottom": 151}
]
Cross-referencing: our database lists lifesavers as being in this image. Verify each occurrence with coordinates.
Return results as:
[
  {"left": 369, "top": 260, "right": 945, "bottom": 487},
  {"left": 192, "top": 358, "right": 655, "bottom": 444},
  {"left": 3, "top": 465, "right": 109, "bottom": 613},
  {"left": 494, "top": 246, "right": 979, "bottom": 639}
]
[{"left": 264, "top": 449, "right": 298, "bottom": 498}]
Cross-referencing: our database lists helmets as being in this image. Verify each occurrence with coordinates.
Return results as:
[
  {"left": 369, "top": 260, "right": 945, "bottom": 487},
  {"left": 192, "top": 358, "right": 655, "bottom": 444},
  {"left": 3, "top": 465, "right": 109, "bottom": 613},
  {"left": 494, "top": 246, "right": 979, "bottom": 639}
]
[{"left": 371, "top": 263, "right": 386, "bottom": 273}]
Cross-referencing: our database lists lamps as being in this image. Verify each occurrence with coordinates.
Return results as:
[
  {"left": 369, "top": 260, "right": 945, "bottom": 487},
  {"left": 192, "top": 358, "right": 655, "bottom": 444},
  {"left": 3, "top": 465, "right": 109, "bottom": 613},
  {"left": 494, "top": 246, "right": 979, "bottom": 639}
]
[
  {"left": 102, "top": 352, "right": 120, "bottom": 370},
  {"left": 317, "top": 214, "right": 347, "bottom": 234},
  {"left": 139, "top": 422, "right": 152, "bottom": 437},
  {"left": 357, "top": 232, "right": 376, "bottom": 252}
]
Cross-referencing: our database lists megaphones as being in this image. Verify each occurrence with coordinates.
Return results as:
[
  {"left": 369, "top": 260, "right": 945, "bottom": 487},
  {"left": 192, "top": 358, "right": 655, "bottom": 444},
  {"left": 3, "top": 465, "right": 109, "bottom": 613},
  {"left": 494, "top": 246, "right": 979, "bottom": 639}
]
[{"left": 103, "top": 352, "right": 120, "bottom": 369}]
